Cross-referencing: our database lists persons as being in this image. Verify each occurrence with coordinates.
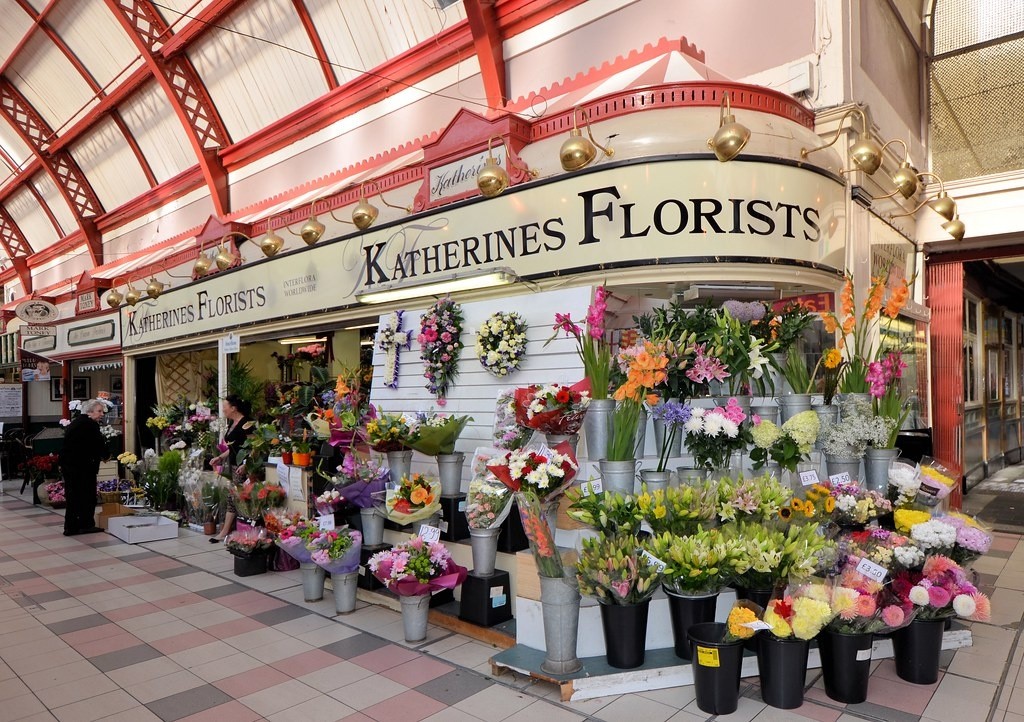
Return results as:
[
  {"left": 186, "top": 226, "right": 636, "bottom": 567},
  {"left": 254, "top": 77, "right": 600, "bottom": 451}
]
[
  {"left": 58, "top": 399, "right": 113, "bottom": 536},
  {"left": 208, "top": 396, "right": 260, "bottom": 544},
  {"left": 37, "top": 361, "right": 49, "bottom": 378}
]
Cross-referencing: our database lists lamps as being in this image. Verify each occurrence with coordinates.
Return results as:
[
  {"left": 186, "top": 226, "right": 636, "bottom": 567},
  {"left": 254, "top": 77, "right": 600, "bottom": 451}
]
[
  {"left": 302, "top": 198, "right": 354, "bottom": 247},
  {"left": 126, "top": 273, "right": 149, "bottom": 307},
  {"left": 840, "top": 138, "right": 919, "bottom": 200},
  {"left": 476, "top": 133, "right": 535, "bottom": 197},
  {"left": 875, "top": 174, "right": 956, "bottom": 222},
  {"left": 706, "top": 92, "right": 752, "bottom": 160},
  {"left": 260, "top": 214, "right": 302, "bottom": 257},
  {"left": 215, "top": 231, "right": 260, "bottom": 271},
  {"left": 278, "top": 321, "right": 383, "bottom": 349},
  {"left": 941, "top": 213, "right": 967, "bottom": 240},
  {"left": 560, "top": 106, "right": 613, "bottom": 172},
  {"left": 798, "top": 109, "right": 887, "bottom": 175},
  {"left": 194, "top": 238, "right": 246, "bottom": 277},
  {"left": 145, "top": 263, "right": 193, "bottom": 300},
  {"left": 106, "top": 277, "right": 135, "bottom": 309},
  {"left": 351, "top": 179, "right": 413, "bottom": 230}
]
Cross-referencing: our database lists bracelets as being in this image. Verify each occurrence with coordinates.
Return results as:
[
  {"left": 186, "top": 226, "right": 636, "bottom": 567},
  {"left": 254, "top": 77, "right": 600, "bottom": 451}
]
[{"left": 218, "top": 456, "right": 223, "bottom": 462}]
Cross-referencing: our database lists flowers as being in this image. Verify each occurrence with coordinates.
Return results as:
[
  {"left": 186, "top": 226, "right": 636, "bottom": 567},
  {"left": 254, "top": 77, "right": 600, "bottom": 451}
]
[{"left": 22, "top": 261, "right": 996, "bottom": 642}]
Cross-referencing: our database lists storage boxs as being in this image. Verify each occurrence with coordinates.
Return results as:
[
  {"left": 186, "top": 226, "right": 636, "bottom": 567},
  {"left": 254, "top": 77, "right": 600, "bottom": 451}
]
[
  {"left": 108, "top": 515, "right": 181, "bottom": 544},
  {"left": 95, "top": 502, "right": 139, "bottom": 528}
]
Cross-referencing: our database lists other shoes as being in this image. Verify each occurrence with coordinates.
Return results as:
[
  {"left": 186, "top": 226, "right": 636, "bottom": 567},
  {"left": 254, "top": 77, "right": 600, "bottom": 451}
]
[
  {"left": 84, "top": 527, "right": 105, "bottom": 533},
  {"left": 209, "top": 538, "right": 224, "bottom": 542}
]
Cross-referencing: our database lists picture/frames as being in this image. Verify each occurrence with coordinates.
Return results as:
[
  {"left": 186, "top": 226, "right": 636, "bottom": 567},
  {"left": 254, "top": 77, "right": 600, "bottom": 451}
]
[
  {"left": 110, "top": 375, "right": 123, "bottom": 391},
  {"left": 50, "top": 376, "right": 92, "bottom": 402}
]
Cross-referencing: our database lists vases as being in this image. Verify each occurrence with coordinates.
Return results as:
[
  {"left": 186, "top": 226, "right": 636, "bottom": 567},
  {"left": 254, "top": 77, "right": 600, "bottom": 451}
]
[{"left": 36, "top": 358, "right": 957, "bottom": 713}]
